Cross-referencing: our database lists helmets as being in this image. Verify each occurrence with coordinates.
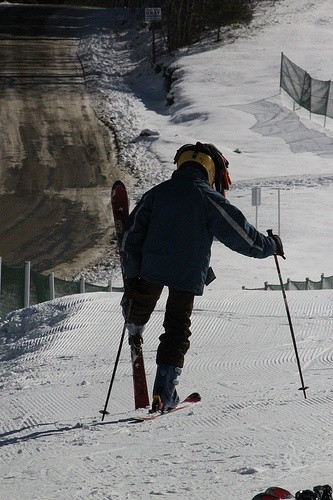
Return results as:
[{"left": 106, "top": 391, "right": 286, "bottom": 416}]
[{"left": 175, "top": 143, "right": 216, "bottom": 186}]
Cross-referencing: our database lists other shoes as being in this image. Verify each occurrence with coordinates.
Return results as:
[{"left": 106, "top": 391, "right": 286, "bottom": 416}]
[
  {"left": 125, "top": 321, "right": 146, "bottom": 340},
  {"left": 149, "top": 366, "right": 183, "bottom": 409}
]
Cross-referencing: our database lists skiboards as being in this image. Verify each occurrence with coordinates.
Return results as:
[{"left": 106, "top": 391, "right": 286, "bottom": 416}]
[
  {"left": 110, "top": 180, "right": 151, "bottom": 411},
  {"left": 130, "top": 392, "right": 201, "bottom": 421}
]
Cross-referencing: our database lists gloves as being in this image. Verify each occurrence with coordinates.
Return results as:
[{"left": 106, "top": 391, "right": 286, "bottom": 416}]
[{"left": 269, "top": 234, "right": 287, "bottom": 258}]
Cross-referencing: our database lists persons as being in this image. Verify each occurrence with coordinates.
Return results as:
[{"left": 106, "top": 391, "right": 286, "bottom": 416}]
[{"left": 120, "top": 142, "right": 284, "bottom": 413}]
